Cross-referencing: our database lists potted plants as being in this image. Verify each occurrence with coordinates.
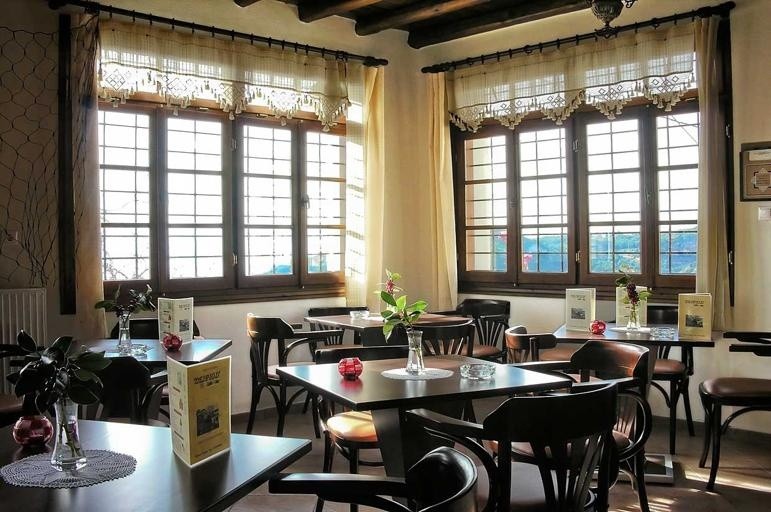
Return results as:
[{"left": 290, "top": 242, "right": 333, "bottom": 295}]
[{"left": 94, "top": 282, "right": 156, "bottom": 350}]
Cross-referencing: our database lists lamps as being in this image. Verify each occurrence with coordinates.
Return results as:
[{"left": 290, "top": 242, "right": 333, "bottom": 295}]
[{"left": 586, "top": 1, "right": 636, "bottom": 38}]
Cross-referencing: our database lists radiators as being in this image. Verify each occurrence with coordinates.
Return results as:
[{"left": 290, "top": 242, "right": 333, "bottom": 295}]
[{"left": 0, "top": 289, "right": 46, "bottom": 397}]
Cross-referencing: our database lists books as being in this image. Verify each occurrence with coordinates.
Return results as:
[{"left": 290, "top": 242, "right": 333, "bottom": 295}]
[
  {"left": 676, "top": 292, "right": 714, "bottom": 341},
  {"left": 615, "top": 285, "right": 648, "bottom": 329},
  {"left": 565, "top": 284, "right": 599, "bottom": 334}
]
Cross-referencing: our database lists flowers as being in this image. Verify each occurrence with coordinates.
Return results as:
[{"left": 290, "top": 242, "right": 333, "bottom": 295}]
[
  {"left": 614, "top": 270, "right": 650, "bottom": 307},
  {"left": 370, "top": 268, "right": 402, "bottom": 296}
]
